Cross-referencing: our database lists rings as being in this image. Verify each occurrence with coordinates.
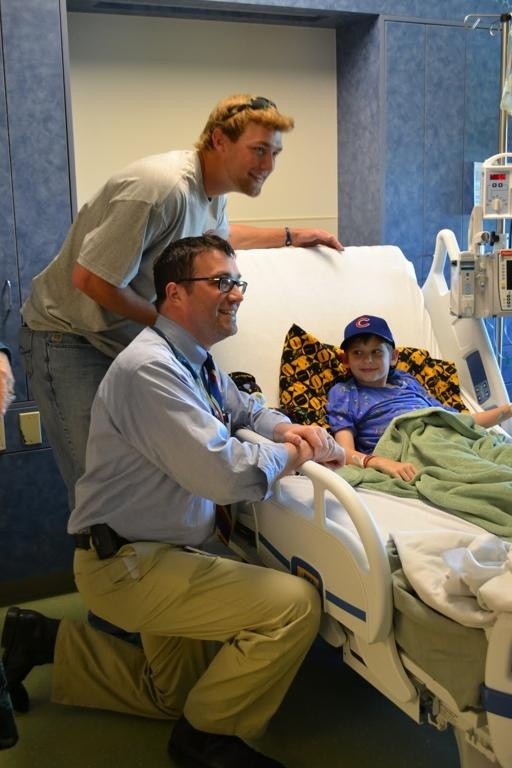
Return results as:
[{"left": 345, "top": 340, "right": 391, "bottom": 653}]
[{"left": 326, "top": 436, "right": 334, "bottom": 441}]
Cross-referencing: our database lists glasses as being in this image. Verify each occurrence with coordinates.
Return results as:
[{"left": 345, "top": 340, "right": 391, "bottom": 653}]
[
  {"left": 223, "top": 97, "right": 275, "bottom": 120},
  {"left": 174, "top": 278, "right": 247, "bottom": 295}
]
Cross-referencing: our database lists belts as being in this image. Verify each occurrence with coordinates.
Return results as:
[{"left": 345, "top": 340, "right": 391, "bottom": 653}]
[{"left": 74, "top": 533, "right": 91, "bottom": 550}]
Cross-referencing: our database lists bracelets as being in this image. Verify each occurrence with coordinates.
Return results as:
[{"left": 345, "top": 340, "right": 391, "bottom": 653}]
[
  {"left": 365, "top": 456, "right": 377, "bottom": 468},
  {"left": 363, "top": 454, "right": 372, "bottom": 466},
  {"left": 284, "top": 226, "right": 293, "bottom": 248}
]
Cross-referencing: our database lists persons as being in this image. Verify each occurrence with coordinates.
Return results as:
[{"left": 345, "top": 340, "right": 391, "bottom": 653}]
[
  {"left": 325, "top": 314, "right": 512, "bottom": 485},
  {"left": 16, "top": 91, "right": 345, "bottom": 528},
  {"left": 1, "top": 235, "right": 348, "bottom": 765},
  {"left": 1, "top": 272, "right": 19, "bottom": 752}
]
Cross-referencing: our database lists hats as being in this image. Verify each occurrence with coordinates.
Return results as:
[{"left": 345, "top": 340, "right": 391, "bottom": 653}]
[{"left": 340, "top": 315, "right": 394, "bottom": 350}]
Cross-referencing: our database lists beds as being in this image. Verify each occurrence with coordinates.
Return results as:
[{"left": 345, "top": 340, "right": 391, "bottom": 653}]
[{"left": 197, "top": 226, "right": 512, "bottom": 766}]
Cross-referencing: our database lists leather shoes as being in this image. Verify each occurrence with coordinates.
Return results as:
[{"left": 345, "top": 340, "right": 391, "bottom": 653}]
[
  {"left": 168, "top": 714, "right": 285, "bottom": 768},
  {"left": 1, "top": 607, "right": 46, "bottom": 712}
]
